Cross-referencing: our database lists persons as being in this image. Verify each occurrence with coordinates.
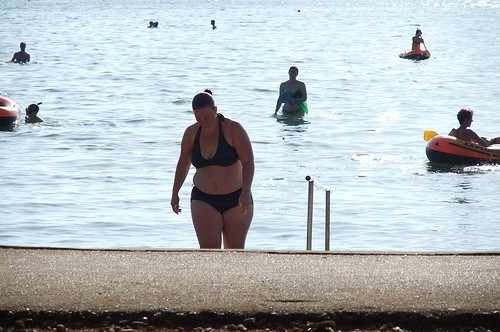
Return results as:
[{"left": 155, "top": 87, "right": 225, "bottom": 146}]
[
  {"left": 210, "top": 19, "right": 216, "bottom": 30},
  {"left": 24, "top": 104, "right": 44, "bottom": 123},
  {"left": 10, "top": 41, "right": 31, "bottom": 64},
  {"left": 153, "top": 21, "right": 160, "bottom": 27},
  {"left": 411, "top": 29, "right": 424, "bottom": 52},
  {"left": 171, "top": 89, "right": 255, "bottom": 251},
  {"left": 147, "top": 21, "right": 153, "bottom": 27},
  {"left": 272, "top": 65, "right": 307, "bottom": 118},
  {"left": 447, "top": 108, "right": 500, "bottom": 148}
]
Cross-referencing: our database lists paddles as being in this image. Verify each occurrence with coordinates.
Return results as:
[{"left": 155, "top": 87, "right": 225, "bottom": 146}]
[
  {"left": 417, "top": 28, "right": 428, "bottom": 51},
  {"left": 423, "top": 130, "right": 500, "bottom": 144}
]
